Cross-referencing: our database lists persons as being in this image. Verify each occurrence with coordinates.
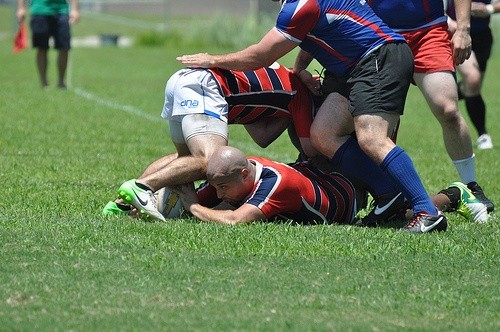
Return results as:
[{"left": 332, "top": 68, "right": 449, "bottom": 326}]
[
  {"left": 175, "top": 0, "right": 500, "bottom": 233},
  {"left": 17, "top": 0, "right": 80, "bottom": 90},
  {"left": 103, "top": 60, "right": 325, "bottom": 222}
]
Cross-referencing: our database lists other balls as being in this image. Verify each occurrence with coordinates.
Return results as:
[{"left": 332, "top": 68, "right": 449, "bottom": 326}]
[{"left": 152, "top": 186, "right": 183, "bottom": 218}]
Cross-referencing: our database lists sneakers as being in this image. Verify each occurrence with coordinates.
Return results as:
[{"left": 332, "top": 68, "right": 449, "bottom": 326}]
[
  {"left": 466, "top": 183, "right": 494, "bottom": 213},
  {"left": 117, "top": 178, "right": 166, "bottom": 223},
  {"left": 477, "top": 134, "right": 493, "bottom": 149},
  {"left": 449, "top": 181, "right": 487, "bottom": 224},
  {"left": 101, "top": 200, "right": 141, "bottom": 217},
  {"left": 400, "top": 211, "right": 448, "bottom": 233},
  {"left": 361, "top": 191, "right": 410, "bottom": 228}
]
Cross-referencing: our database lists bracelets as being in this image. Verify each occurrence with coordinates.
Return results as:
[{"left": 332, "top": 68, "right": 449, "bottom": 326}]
[{"left": 486, "top": 4, "right": 495, "bottom": 14}]
